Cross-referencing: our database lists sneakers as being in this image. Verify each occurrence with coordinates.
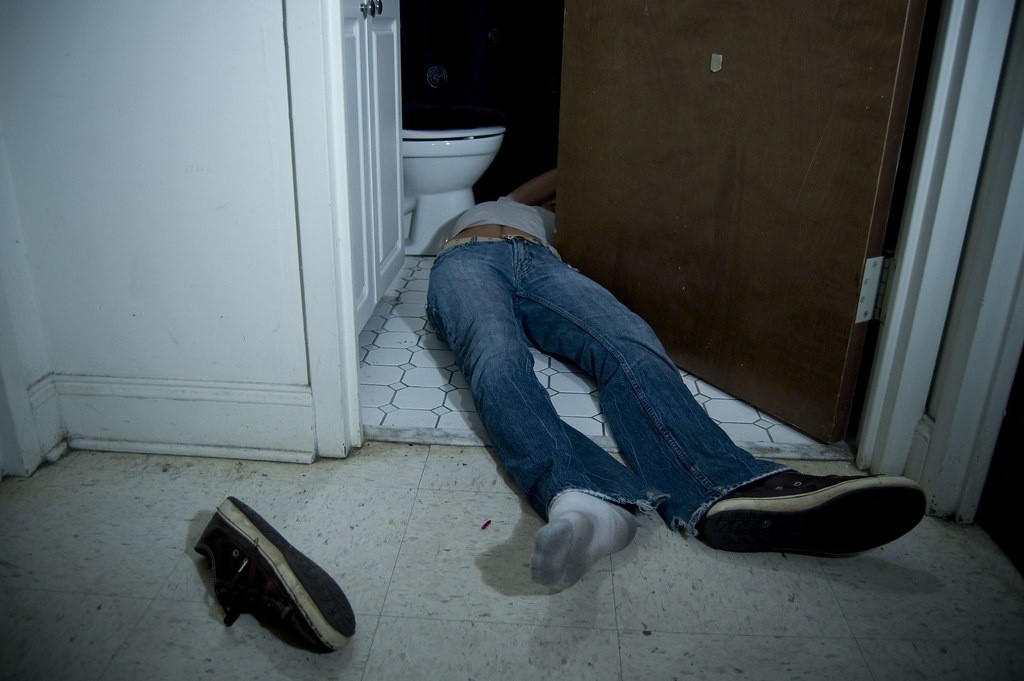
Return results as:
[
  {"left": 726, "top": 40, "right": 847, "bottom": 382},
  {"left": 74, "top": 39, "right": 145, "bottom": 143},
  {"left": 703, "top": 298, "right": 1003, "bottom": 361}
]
[
  {"left": 194, "top": 496, "right": 356, "bottom": 651},
  {"left": 702, "top": 469, "right": 927, "bottom": 556}
]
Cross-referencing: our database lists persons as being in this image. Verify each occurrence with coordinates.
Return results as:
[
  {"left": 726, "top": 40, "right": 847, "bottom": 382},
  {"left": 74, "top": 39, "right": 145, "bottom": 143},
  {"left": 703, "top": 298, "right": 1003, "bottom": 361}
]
[{"left": 425, "top": 164, "right": 928, "bottom": 597}]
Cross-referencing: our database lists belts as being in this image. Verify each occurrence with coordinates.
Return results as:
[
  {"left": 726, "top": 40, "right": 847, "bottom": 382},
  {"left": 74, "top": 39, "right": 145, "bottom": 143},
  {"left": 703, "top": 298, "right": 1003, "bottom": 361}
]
[{"left": 437, "top": 234, "right": 541, "bottom": 255}]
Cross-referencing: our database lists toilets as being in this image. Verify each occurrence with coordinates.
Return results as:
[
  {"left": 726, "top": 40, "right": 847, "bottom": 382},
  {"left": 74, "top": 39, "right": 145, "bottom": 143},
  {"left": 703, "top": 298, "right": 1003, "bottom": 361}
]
[{"left": 400, "top": 125, "right": 507, "bottom": 256}]
